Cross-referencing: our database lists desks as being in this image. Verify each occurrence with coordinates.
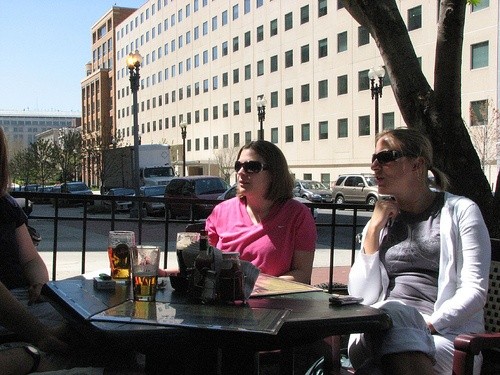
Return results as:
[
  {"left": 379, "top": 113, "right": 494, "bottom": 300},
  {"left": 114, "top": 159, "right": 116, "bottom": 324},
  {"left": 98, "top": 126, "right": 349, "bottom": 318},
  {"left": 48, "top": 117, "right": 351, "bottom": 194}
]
[{"left": 40, "top": 272, "right": 393, "bottom": 375}]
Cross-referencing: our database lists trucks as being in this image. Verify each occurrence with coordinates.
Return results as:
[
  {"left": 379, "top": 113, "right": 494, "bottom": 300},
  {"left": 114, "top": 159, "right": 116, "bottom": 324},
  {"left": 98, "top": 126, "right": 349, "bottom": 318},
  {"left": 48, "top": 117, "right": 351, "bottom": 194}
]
[{"left": 102, "top": 144, "right": 176, "bottom": 189}]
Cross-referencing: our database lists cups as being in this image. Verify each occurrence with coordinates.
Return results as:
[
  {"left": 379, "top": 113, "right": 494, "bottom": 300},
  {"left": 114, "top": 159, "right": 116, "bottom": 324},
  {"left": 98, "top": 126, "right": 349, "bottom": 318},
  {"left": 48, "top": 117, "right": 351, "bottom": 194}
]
[
  {"left": 176, "top": 232, "right": 201, "bottom": 281},
  {"left": 108, "top": 230, "right": 136, "bottom": 277},
  {"left": 128, "top": 245, "right": 160, "bottom": 302}
]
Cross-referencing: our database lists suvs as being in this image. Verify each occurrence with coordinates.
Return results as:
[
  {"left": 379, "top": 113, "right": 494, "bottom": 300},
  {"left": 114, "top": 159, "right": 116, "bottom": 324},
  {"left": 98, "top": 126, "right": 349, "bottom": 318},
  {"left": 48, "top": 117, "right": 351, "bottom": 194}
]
[
  {"left": 332, "top": 173, "right": 391, "bottom": 211},
  {"left": 61, "top": 180, "right": 94, "bottom": 206},
  {"left": 24, "top": 184, "right": 40, "bottom": 191}
]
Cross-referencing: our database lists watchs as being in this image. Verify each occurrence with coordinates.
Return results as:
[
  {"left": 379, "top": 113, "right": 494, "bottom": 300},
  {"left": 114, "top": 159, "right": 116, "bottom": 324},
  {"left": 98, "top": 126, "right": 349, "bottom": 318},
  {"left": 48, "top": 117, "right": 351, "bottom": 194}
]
[{"left": 23, "top": 345, "right": 40, "bottom": 373}]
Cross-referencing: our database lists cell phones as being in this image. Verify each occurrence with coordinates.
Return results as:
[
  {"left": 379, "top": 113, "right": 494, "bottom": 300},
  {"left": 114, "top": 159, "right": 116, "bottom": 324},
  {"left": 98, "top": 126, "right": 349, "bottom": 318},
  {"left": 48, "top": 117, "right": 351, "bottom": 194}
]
[{"left": 329, "top": 296, "right": 363, "bottom": 305}]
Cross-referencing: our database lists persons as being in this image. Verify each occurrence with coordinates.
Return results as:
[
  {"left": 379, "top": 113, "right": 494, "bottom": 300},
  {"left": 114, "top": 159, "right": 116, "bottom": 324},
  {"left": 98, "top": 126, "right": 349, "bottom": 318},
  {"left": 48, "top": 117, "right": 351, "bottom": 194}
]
[
  {"left": 347, "top": 128, "right": 491, "bottom": 375},
  {"left": 0, "top": 125, "right": 66, "bottom": 330},
  {"left": 0, "top": 282, "right": 78, "bottom": 375},
  {"left": 140, "top": 139, "right": 316, "bottom": 286}
]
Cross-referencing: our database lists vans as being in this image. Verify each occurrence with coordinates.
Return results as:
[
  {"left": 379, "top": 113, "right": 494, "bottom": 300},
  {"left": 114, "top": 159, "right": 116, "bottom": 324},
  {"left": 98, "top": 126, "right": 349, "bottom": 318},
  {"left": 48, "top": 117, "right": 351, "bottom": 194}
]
[{"left": 164, "top": 176, "right": 235, "bottom": 220}]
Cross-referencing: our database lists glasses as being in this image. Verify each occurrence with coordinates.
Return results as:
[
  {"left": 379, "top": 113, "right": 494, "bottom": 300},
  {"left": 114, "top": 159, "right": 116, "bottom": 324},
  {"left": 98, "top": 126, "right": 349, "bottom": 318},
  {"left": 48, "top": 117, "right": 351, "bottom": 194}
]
[
  {"left": 234, "top": 161, "right": 268, "bottom": 174},
  {"left": 371, "top": 150, "right": 409, "bottom": 164}
]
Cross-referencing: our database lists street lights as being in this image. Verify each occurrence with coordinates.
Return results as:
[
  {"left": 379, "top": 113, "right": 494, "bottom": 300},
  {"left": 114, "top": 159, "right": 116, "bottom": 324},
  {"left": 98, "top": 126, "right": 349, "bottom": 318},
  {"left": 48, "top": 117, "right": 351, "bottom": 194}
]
[
  {"left": 127, "top": 50, "right": 142, "bottom": 187},
  {"left": 368, "top": 66, "right": 385, "bottom": 136},
  {"left": 256, "top": 98, "right": 266, "bottom": 140},
  {"left": 179, "top": 121, "right": 188, "bottom": 176}
]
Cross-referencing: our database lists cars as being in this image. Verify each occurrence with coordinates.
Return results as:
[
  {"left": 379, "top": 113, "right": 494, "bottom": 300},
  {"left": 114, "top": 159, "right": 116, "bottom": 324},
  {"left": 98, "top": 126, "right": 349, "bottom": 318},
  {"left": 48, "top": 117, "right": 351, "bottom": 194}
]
[
  {"left": 217, "top": 183, "right": 317, "bottom": 222},
  {"left": 132, "top": 185, "right": 168, "bottom": 213},
  {"left": 295, "top": 179, "right": 333, "bottom": 202},
  {"left": 54, "top": 184, "right": 60, "bottom": 189},
  {"left": 103, "top": 188, "right": 134, "bottom": 209},
  {"left": 36, "top": 187, "right": 54, "bottom": 192}
]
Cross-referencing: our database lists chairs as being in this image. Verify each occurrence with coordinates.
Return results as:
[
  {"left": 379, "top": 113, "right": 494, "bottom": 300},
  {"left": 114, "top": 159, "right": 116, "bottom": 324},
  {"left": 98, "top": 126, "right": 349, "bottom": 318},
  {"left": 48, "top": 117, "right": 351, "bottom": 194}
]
[{"left": 334, "top": 237, "right": 500, "bottom": 375}]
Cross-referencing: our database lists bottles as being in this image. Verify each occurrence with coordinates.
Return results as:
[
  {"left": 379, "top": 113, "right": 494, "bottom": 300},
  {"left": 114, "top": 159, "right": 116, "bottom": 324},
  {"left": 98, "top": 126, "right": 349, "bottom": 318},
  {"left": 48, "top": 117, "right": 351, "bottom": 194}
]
[{"left": 195, "top": 230, "right": 240, "bottom": 300}]
